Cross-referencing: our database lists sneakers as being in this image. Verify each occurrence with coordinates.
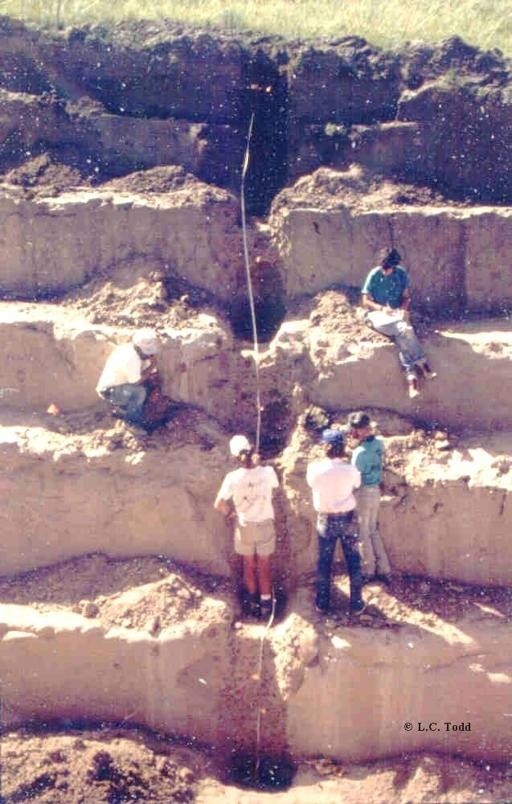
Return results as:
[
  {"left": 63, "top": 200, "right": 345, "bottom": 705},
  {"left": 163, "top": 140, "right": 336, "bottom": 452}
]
[
  {"left": 416, "top": 357, "right": 438, "bottom": 381},
  {"left": 378, "top": 572, "right": 392, "bottom": 584},
  {"left": 405, "top": 374, "right": 421, "bottom": 400},
  {"left": 243, "top": 593, "right": 259, "bottom": 613},
  {"left": 362, "top": 575, "right": 376, "bottom": 586},
  {"left": 314, "top": 600, "right": 329, "bottom": 612},
  {"left": 261, "top": 592, "right": 285, "bottom": 615},
  {"left": 349, "top": 603, "right": 368, "bottom": 615}
]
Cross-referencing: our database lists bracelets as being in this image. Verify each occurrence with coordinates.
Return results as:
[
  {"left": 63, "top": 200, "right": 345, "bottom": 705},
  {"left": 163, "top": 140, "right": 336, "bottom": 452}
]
[{"left": 226, "top": 508, "right": 232, "bottom": 517}]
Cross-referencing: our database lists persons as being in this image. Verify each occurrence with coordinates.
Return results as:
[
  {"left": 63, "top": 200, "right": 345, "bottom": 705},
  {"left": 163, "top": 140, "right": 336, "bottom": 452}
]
[
  {"left": 362, "top": 247, "right": 436, "bottom": 397},
  {"left": 96, "top": 328, "right": 159, "bottom": 421},
  {"left": 305, "top": 430, "right": 367, "bottom": 615},
  {"left": 349, "top": 412, "right": 393, "bottom": 585},
  {"left": 213, "top": 434, "right": 280, "bottom": 618}
]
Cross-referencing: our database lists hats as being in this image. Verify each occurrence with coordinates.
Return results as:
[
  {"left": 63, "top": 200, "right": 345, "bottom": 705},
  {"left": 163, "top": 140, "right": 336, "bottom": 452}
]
[
  {"left": 227, "top": 434, "right": 254, "bottom": 454},
  {"left": 133, "top": 326, "right": 162, "bottom": 356},
  {"left": 345, "top": 410, "right": 371, "bottom": 427},
  {"left": 323, "top": 430, "right": 345, "bottom": 443}
]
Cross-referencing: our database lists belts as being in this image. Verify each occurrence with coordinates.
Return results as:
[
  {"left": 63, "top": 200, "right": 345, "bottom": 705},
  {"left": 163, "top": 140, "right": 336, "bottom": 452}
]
[{"left": 319, "top": 510, "right": 356, "bottom": 517}]
